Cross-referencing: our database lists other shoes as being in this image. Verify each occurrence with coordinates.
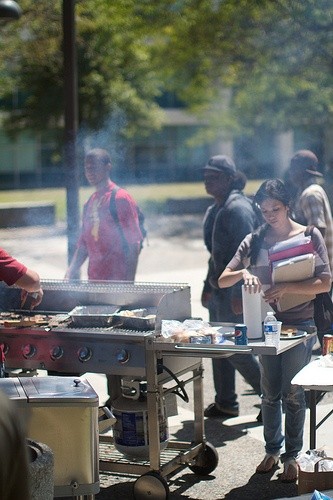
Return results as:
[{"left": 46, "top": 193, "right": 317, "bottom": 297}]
[{"left": 203, "top": 402, "right": 236, "bottom": 418}]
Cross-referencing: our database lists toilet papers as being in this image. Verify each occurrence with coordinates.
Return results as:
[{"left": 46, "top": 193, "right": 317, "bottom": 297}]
[{"left": 241, "top": 284, "right": 277, "bottom": 339}]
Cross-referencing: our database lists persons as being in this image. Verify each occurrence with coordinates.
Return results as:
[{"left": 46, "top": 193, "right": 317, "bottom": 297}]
[
  {"left": 284, "top": 151, "right": 333, "bottom": 351},
  {"left": 64, "top": 148, "right": 142, "bottom": 406},
  {"left": 215, "top": 178, "right": 332, "bottom": 482},
  {"left": 201, "top": 155, "right": 262, "bottom": 422},
  {"left": 0, "top": 248, "right": 43, "bottom": 310}
]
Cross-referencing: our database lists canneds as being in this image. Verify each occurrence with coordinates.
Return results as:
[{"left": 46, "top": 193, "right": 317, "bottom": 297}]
[{"left": 234, "top": 324, "right": 248, "bottom": 345}]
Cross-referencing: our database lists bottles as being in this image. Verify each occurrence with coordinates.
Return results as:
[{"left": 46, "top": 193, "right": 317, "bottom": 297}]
[{"left": 263, "top": 312, "right": 279, "bottom": 351}]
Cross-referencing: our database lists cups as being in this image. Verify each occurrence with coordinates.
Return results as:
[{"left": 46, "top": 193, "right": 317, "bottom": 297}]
[{"left": 276, "top": 321, "right": 282, "bottom": 340}]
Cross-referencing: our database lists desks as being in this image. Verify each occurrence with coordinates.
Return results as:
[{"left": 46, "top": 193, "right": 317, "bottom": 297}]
[{"left": 290, "top": 356, "right": 333, "bottom": 449}]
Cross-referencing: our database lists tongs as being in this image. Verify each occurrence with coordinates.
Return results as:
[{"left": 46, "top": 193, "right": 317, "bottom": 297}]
[{"left": 19, "top": 292, "right": 39, "bottom": 316}]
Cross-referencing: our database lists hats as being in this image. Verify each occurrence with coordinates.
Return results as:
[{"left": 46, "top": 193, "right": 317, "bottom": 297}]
[
  {"left": 290, "top": 149, "right": 325, "bottom": 179},
  {"left": 198, "top": 155, "right": 237, "bottom": 177}
]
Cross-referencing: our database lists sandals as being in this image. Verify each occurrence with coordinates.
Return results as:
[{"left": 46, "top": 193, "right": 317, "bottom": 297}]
[
  {"left": 255, "top": 451, "right": 279, "bottom": 473},
  {"left": 280, "top": 459, "right": 299, "bottom": 481}
]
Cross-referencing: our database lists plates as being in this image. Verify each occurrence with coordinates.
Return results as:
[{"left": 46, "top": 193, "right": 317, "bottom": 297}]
[{"left": 278, "top": 330, "right": 307, "bottom": 339}]
